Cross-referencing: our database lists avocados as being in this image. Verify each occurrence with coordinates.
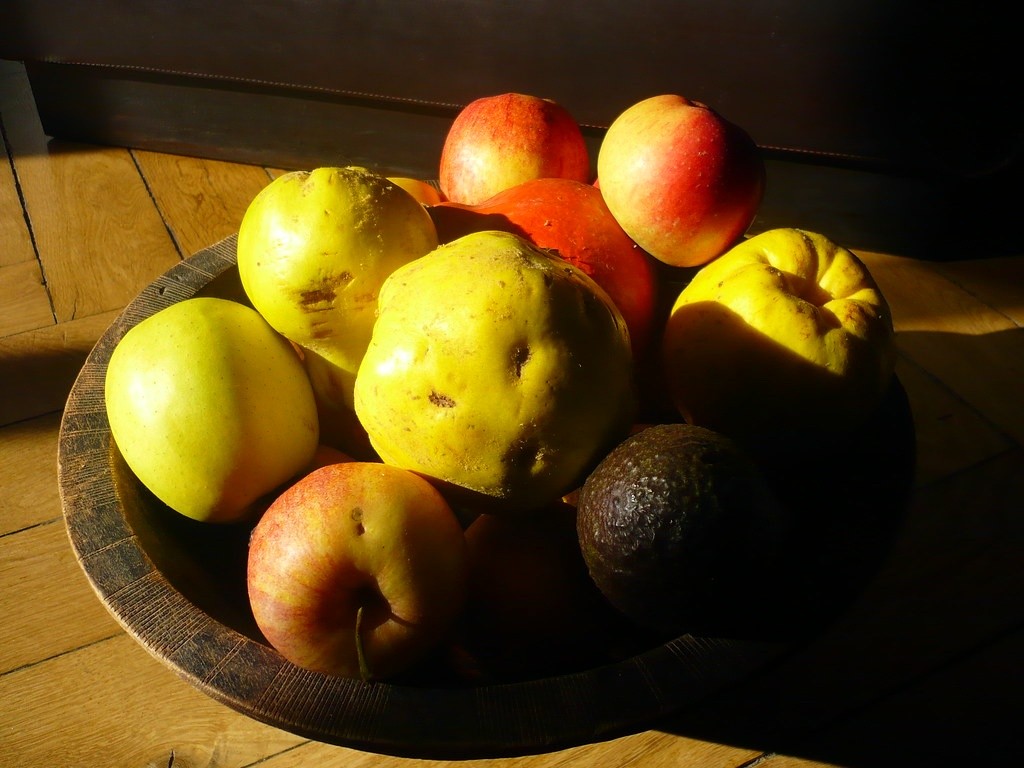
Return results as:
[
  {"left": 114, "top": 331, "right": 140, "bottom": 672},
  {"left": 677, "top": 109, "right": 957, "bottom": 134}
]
[{"left": 577, "top": 423, "right": 762, "bottom": 618}]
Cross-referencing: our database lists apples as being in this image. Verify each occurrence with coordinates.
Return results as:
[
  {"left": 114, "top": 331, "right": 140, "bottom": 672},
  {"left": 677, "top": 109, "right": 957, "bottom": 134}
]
[{"left": 104, "top": 95, "right": 893, "bottom": 680}]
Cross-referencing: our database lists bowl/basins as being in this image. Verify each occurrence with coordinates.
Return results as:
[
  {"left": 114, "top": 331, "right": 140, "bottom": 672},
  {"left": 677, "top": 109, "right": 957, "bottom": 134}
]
[{"left": 54, "top": 232, "right": 915, "bottom": 762}]
